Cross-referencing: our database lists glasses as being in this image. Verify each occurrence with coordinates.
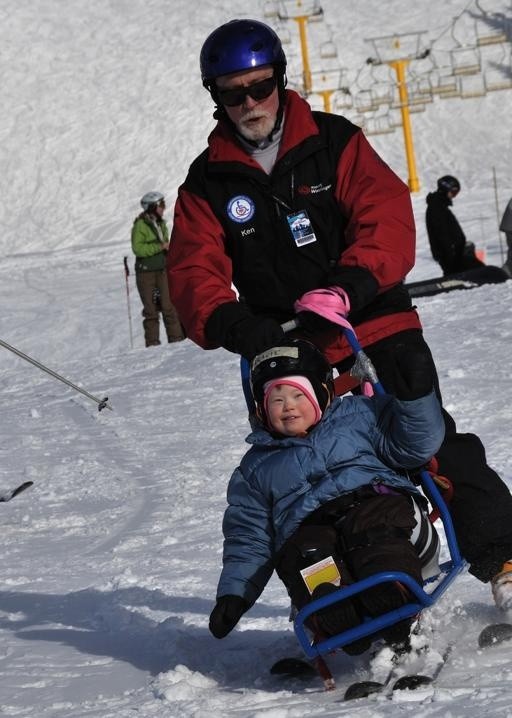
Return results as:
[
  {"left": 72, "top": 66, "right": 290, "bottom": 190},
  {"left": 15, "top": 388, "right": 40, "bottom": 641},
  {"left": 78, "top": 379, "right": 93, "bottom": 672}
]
[{"left": 212, "top": 77, "right": 278, "bottom": 107}]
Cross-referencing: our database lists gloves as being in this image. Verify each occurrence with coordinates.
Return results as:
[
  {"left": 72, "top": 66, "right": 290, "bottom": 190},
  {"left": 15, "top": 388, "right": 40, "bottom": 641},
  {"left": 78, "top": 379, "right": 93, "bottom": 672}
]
[
  {"left": 295, "top": 291, "right": 345, "bottom": 350},
  {"left": 236, "top": 319, "right": 284, "bottom": 360},
  {"left": 386, "top": 343, "right": 435, "bottom": 401},
  {"left": 208, "top": 595, "right": 248, "bottom": 639}
]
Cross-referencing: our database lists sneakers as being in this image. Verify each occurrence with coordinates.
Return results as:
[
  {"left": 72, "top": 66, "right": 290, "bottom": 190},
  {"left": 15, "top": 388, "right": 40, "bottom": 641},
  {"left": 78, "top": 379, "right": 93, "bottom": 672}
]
[{"left": 491, "top": 562, "right": 512, "bottom": 614}]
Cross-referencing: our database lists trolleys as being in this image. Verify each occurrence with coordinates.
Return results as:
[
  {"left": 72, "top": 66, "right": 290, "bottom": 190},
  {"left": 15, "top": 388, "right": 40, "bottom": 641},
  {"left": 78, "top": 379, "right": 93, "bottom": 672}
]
[{"left": 239, "top": 316, "right": 479, "bottom": 698}]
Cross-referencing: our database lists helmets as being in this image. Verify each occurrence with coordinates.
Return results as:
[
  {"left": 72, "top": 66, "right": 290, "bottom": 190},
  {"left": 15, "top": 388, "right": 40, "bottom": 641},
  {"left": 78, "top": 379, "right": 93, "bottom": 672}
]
[
  {"left": 437, "top": 175, "right": 461, "bottom": 197},
  {"left": 249, "top": 339, "right": 331, "bottom": 402},
  {"left": 140, "top": 192, "right": 165, "bottom": 212},
  {"left": 202, "top": 18, "right": 286, "bottom": 80}
]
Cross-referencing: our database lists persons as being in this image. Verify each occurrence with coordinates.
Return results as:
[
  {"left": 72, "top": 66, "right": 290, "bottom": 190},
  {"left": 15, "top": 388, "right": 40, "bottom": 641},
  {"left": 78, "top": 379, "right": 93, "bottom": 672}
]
[
  {"left": 130, "top": 191, "right": 186, "bottom": 349},
  {"left": 424, "top": 170, "right": 512, "bottom": 285},
  {"left": 204, "top": 336, "right": 450, "bottom": 655},
  {"left": 163, "top": 20, "right": 512, "bottom": 615}
]
